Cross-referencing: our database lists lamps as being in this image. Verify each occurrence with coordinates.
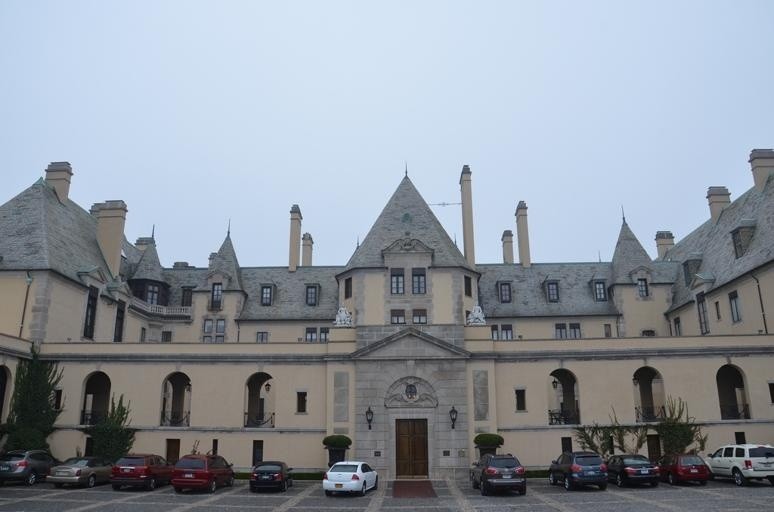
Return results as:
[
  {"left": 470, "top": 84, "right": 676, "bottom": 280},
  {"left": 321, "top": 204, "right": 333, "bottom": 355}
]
[
  {"left": 450, "top": 406, "right": 458, "bottom": 429},
  {"left": 366, "top": 406, "right": 373, "bottom": 429}
]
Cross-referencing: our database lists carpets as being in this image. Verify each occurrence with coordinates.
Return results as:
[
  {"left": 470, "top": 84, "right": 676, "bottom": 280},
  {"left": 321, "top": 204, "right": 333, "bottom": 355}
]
[{"left": 393, "top": 481, "right": 438, "bottom": 499}]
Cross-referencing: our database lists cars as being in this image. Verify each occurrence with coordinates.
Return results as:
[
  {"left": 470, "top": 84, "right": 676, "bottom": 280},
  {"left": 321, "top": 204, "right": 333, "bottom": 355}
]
[
  {"left": 322, "top": 461, "right": 379, "bottom": 497},
  {"left": 248, "top": 460, "right": 294, "bottom": 492},
  {"left": 469, "top": 452, "right": 527, "bottom": 497},
  {"left": 548, "top": 451, "right": 710, "bottom": 492},
  {"left": 0, "top": 447, "right": 236, "bottom": 495}
]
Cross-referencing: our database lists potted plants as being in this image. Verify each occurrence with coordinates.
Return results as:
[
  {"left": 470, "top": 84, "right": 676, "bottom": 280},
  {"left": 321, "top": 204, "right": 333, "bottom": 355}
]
[
  {"left": 322, "top": 435, "right": 352, "bottom": 469},
  {"left": 474, "top": 434, "right": 504, "bottom": 457}
]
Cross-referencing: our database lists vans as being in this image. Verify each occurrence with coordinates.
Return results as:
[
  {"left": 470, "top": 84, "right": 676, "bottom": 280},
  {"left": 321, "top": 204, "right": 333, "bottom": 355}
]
[{"left": 702, "top": 443, "right": 774, "bottom": 487}]
